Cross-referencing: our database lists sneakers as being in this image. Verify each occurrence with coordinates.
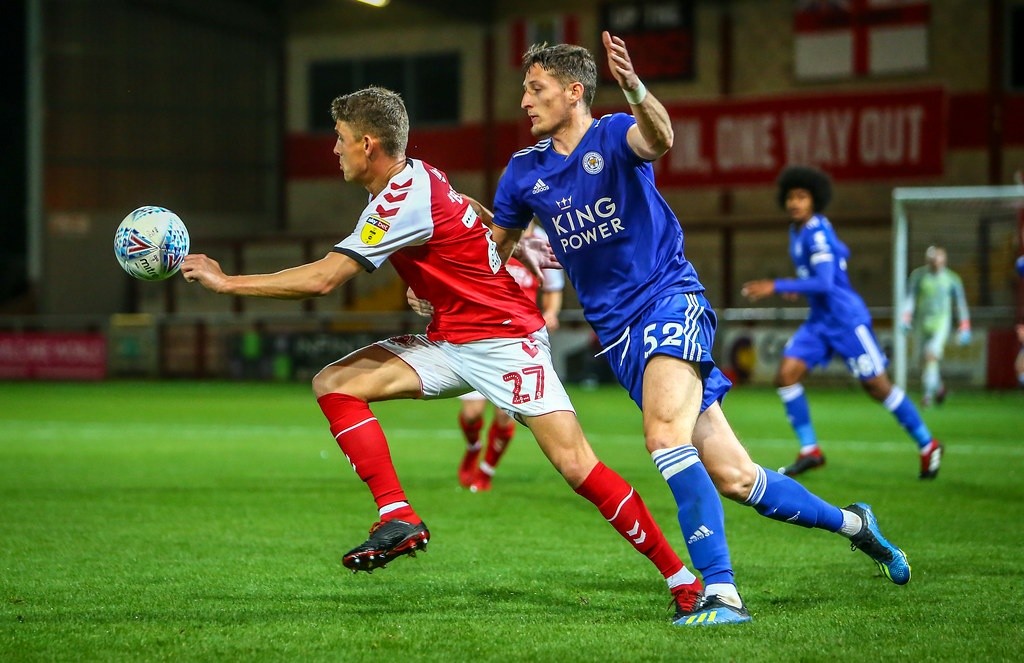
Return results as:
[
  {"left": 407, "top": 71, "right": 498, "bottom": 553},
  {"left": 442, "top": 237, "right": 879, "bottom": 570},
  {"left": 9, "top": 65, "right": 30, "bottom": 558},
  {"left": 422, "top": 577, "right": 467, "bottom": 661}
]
[
  {"left": 470, "top": 469, "right": 491, "bottom": 492},
  {"left": 919, "top": 440, "right": 944, "bottom": 479},
  {"left": 666, "top": 576, "right": 703, "bottom": 620},
  {"left": 343, "top": 506, "right": 430, "bottom": 574},
  {"left": 842, "top": 502, "right": 911, "bottom": 585},
  {"left": 778, "top": 448, "right": 825, "bottom": 476},
  {"left": 673, "top": 593, "right": 754, "bottom": 628},
  {"left": 459, "top": 447, "right": 481, "bottom": 487}
]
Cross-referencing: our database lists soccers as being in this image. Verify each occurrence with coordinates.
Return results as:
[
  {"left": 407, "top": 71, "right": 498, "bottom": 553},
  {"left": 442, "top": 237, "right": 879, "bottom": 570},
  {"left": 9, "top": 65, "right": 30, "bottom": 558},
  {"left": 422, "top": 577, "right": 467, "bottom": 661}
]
[{"left": 112, "top": 204, "right": 191, "bottom": 282}]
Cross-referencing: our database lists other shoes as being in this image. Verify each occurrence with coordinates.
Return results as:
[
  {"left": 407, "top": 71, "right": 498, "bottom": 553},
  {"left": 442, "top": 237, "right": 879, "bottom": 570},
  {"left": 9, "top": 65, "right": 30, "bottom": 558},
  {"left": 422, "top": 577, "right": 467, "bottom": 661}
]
[
  {"left": 922, "top": 392, "right": 932, "bottom": 412},
  {"left": 935, "top": 382, "right": 947, "bottom": 405}
]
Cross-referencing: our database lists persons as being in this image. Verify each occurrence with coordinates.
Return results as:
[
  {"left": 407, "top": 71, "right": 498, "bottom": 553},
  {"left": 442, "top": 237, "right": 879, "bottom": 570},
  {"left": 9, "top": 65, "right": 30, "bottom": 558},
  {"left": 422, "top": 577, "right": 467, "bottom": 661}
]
[
  {"left": 741, "top": 165, "right": 943, "bottom": 479},
  {"left": 407, "top": 31, "right": 912, "bottom": 627},
  {"left": 457, "top": 164, "right": 567, "bottom": 490},
  {"left": 178, "top": 85, "right": 706, "bottom": 621},
  {"left": 1015, "top": 255, "right": 1024, "bottom": 383},
  {"left": 900, "top": 242, "right": 971, "bottom": 408}
]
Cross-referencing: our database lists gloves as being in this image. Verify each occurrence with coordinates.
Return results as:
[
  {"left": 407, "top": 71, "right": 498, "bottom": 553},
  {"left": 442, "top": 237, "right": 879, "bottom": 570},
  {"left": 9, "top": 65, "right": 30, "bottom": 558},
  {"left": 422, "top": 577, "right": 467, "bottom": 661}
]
[
  {"left": 901, "top": 321, "right": 911, "bottom": 337},
  {"left": 956, "top": 328, "right": 972, "bottom": 349}
]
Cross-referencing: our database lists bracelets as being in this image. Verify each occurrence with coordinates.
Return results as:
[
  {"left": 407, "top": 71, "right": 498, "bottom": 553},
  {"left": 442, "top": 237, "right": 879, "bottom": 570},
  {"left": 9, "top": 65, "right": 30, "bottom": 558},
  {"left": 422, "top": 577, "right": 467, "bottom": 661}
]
[{"left": 622, "top": 81, "right": 648, "bottom": 105}]
